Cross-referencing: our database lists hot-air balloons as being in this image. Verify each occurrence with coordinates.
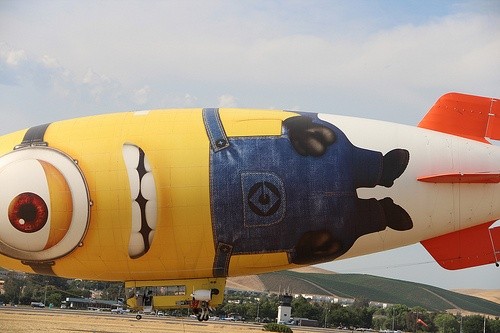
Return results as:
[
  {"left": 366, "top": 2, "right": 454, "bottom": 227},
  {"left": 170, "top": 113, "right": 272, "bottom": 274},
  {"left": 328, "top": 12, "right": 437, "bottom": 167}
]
[{"left": 1, "top": 91, "right": 498, "bottom": 321}]
